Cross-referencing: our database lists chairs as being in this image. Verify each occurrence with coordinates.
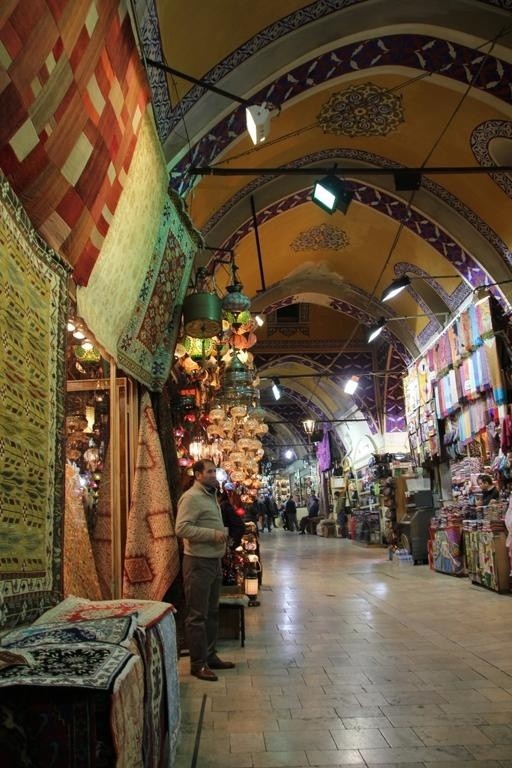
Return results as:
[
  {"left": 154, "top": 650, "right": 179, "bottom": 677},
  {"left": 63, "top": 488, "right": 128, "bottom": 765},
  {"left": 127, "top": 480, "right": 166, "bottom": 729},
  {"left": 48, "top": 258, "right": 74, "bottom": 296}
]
[
  {"left": 283, "top": 526, "right": 287, "bottom": 530},
  {"left": 299, "top": 531, "right": 305, "bottom": 535},
  {"left": 341, "top": 535, "right": 346, "bottom": 538},
  {"left": 274, "top": 525, "right": 278, "bottom": 528},
  {"left": 259, "top": 529, "right": 264, "bottom": 533},
  {"left": 268, "top": 529, "right": 272, "bottom": 532},
  {"left": 290, "top": 530, "right": 294, "bottom": 531},
  {"left": 296, "top": 528, "right": 299, "bottom": 531}
]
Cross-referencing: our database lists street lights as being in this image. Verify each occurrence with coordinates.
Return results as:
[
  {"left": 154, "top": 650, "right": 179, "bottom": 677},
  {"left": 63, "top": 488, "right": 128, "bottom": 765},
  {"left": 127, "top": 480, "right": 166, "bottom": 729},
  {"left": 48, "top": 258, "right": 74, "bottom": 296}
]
[{"left": 344, "top": 506, "right": 352, "bottom": 514}]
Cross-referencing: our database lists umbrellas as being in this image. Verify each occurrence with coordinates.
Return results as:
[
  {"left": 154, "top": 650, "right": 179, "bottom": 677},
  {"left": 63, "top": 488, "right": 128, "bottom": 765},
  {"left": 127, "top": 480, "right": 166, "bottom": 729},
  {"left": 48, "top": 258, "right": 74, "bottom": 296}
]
[
  {"left": 207, "top": 653, "right": 234, "bottom": 669},
  {"left": 191, "top": 663, "right": 218, "bottom": 681}
]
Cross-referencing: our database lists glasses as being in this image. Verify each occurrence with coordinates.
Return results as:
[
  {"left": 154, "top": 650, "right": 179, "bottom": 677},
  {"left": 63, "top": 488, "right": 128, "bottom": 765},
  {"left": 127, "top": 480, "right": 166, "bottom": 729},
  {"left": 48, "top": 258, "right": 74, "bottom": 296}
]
[
  {"left": 312, "top": 174, "right": 353, "bottom": 216},
  {"left": 379, "top": 276, "right": 461, "bottom": 304},
  {"left": 300, "top": 416, "right": 371, "bottom": 445},
  {"left": 270, "top": 376, "right": 281, "bottom": 400},
  {"left": 367, "top": 311, "right": 450, "bottom": 344},
  {"left": 140, "top": 57, "right": 281, "bottom": 146},
  {"left": 344, "top": 370, "right": 401, "bottom": 395}
]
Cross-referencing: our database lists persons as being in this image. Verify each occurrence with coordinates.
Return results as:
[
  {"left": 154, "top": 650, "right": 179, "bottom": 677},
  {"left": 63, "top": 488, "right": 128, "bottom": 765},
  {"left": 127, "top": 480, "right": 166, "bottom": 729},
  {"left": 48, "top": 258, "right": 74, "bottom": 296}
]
[
  {"left": 262, "top": 489, "right": 320, "bottom": 535},
  {"left": 475, "top": 474, "right": 501, "bottom": 505},
  {"left": 217, "top": 491, "right": 245, "bottom": 550},
  {"left": 174, "top": 458, "right": 236, "bottom": 680},
  {"left": 334, "top": 491, "right": 345, "bottom": 537}
]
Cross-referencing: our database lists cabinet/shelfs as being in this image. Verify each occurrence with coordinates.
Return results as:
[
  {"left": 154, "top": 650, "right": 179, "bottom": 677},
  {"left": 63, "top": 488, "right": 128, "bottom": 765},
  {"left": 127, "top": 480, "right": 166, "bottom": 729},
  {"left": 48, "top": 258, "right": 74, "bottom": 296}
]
[
  {"left": 392, "top": 547, "right": 413, "bottom": 569},
  {"left": 244, "top": 561, "right": 258, "bottom": 602}
]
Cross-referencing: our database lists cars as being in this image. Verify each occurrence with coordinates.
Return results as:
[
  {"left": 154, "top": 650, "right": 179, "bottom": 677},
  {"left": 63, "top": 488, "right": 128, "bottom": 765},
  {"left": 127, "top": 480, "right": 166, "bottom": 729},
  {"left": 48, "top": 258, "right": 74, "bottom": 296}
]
[{"left": 414, "top": 467, "right": 424, "bottom": 478}]
